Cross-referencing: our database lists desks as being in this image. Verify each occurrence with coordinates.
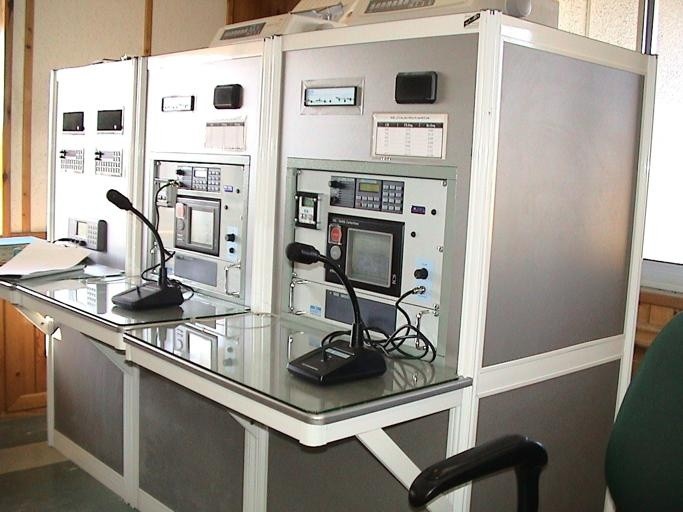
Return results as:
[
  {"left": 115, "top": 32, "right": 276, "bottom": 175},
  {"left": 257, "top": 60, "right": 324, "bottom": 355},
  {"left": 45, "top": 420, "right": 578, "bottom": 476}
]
[{"left": 0, "top": 256, "right": 475, "bottom": 512}]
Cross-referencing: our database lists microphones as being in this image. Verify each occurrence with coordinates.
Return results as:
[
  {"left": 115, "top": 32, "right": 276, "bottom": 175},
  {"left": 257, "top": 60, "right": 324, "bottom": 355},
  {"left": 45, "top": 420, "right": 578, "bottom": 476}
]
[
  {"left": 285, "top": 242, "right": 386, "bottom": 386},
  {"left": 107, "top": 190, "right": 184, "bottom": 311}
]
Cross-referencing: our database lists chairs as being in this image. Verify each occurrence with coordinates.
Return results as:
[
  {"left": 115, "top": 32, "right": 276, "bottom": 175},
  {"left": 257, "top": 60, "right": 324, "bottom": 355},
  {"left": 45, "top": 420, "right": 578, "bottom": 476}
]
[{"left": 402, "top": 307, "right": 682, "bottom": 512}]
